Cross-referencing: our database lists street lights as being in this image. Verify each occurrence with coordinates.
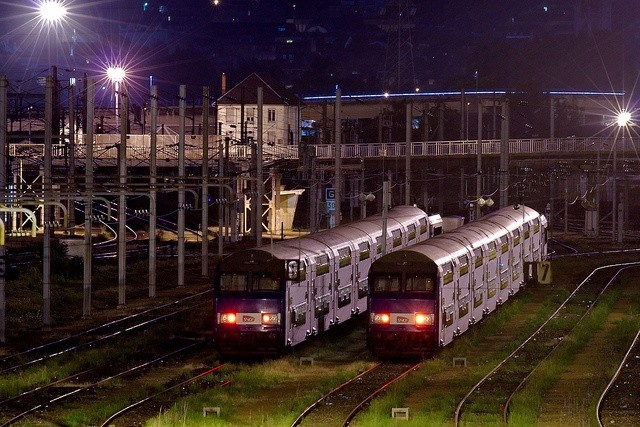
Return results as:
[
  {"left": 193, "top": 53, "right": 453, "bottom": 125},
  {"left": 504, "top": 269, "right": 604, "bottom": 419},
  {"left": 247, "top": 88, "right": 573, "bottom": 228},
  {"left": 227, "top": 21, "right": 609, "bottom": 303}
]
[
  {"left": 611, "top": 111, "right": 631, "bottom": 243},
  {"left": 39, "top": 1, "right": 66, "bottom": 80},
  {"left": 107, "top": 67, "right": 125, "bottom": 133}
]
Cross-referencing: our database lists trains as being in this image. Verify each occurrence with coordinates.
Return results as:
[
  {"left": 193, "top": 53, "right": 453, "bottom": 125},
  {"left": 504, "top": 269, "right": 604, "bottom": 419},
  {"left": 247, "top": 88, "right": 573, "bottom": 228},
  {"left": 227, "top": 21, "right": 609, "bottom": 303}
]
[
  {"left": 366, "top": 203, "right": 549, "bottom": 359},
  {"left": 211, "top": 204, "right": 430, "bottom": 353}
]
